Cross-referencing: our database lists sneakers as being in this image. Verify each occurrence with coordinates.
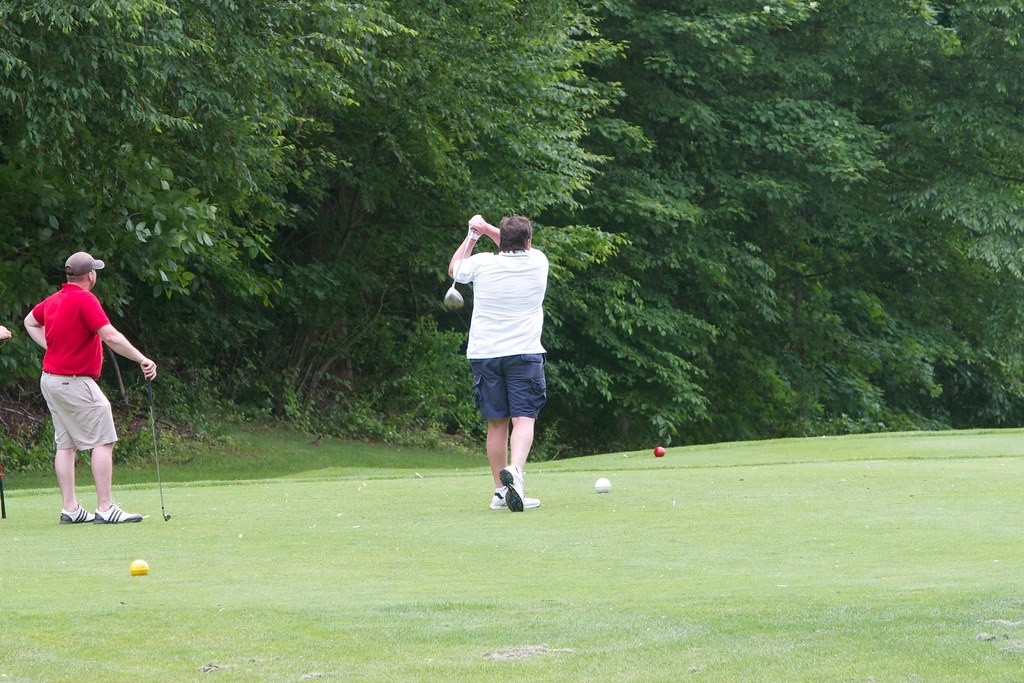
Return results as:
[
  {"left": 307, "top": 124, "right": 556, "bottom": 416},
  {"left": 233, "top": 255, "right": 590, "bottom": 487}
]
[
  {"left": 498, "top": 465, "right": 525, "bottom": 511},
  {"left": 93, "top": 501, "right": 143, "bottom": 524},
  {"left": 59, "top": 499, "right": 95, "bottom": 524},
  {"left": 490, "top": 492, "right": 540, "bottom": 510}
]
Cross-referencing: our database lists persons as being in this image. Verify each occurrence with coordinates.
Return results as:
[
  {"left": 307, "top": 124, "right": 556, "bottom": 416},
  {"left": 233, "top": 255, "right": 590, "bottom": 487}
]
[
  {"left": 448, "top": 215, "right": 549, "bottom": 512},
  {"left": 24, "top": 252, "right": 156, "bottom": 524},
  {"left": 0, "top": 325, "right": 12, "bottom": 339}
]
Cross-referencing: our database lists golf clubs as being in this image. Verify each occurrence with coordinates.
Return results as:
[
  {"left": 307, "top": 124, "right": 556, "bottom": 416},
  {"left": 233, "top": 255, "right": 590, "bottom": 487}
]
[
  {"left": 443, "top": 228, "right": 475, "bottom": 310},
  {"left": 146, "top": 377, "right": 171, "bottom": 521}
]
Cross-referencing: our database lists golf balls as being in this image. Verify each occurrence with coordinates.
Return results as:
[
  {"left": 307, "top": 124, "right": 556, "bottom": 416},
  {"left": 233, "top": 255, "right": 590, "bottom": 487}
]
[
  {"left": 129, "top": 560, "right": 149, "bottom": 576},
  {"left": 654, "top": 446, "right": 665, "bottom": 457},
  {"left": 595, "top": 478, "right": 612, "bottom": 494}
]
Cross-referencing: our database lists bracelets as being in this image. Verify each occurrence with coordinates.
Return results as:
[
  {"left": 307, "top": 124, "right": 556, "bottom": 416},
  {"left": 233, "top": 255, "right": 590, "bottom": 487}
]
[{"left": 466, "top": 231, "right": 480, "bottom": 243}]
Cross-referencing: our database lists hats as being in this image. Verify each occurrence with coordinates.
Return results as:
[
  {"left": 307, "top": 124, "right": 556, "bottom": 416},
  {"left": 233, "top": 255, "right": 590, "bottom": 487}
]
[{"left": 65, "top": 252, "right": 105, "bottom": 275}]
